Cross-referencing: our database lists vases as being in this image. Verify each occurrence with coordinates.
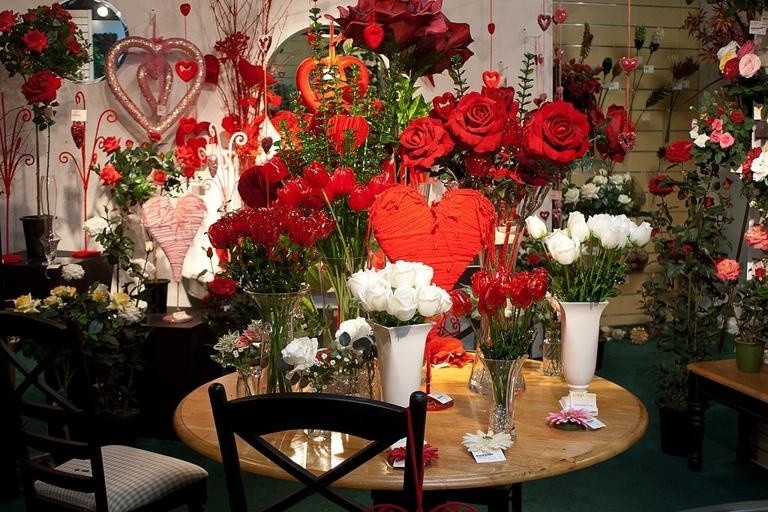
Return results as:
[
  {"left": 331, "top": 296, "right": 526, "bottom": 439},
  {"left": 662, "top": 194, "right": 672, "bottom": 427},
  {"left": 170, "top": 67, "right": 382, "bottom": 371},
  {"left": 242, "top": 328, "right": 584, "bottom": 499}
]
[
  {"left": 141, "top": 278, "right": 170, "bottom": 313},
  {"left": 20, "top": 215, "right": 54, "bottom": 257},
  {"left": 89, "top": 407, "right": 140, "bottom": 446}
]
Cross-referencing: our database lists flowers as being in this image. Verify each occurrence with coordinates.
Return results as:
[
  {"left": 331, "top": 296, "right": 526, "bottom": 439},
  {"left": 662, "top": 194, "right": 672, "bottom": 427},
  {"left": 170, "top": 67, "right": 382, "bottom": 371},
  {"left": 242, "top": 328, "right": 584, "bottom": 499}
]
[
  {"left": 89, "top": 126, "right": 195, "bottom": 280},
  {"left": 11, "top": 213, "right": 158, "bottom": 412},
  {"left": 1, "top": 3, "right": 105, "bottom": 217}
]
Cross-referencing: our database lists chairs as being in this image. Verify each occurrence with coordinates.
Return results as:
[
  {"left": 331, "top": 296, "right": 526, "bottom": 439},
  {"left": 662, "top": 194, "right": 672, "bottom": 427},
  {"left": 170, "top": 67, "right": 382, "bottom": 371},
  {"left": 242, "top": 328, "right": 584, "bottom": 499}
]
[
  {"left": 0, "top": 306, "right": 209, "bottom": 511},
  {"left": 206, "top": 382, "right": 428, "bottom": 512}
]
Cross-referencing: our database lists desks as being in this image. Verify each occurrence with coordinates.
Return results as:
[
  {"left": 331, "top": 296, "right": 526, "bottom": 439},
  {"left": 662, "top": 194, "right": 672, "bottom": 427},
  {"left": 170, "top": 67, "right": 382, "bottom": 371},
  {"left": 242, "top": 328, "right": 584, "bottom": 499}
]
[
  {"left": 685, "top": 357, "right": 768, "bottom": 472},
  {"left": 172, "top": 349, "right": 649, "bottom": 512}
]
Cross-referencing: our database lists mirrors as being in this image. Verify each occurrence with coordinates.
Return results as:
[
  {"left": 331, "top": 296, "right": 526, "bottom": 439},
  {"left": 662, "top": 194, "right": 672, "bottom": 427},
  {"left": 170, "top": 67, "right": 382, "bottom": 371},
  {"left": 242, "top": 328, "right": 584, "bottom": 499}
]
[{"left": 51, "top": 0, "right": 130, "bottom": 85}]
